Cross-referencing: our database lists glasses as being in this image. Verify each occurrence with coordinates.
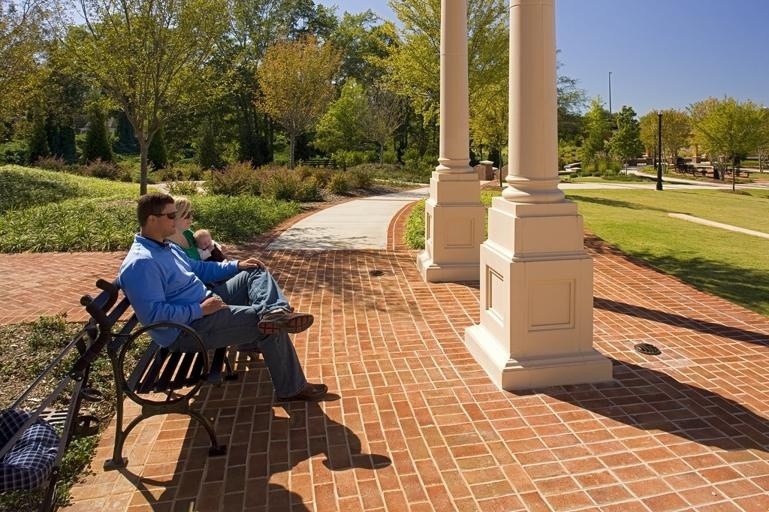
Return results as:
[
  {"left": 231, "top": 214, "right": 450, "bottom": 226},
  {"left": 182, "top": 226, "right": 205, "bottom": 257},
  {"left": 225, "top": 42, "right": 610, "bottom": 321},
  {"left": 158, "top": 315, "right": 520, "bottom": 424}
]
[
  {"left": 182, "top": 211, "right": 191, "bottom": 220},
  {"left": 156, "top": 211, "right": 178, "bottom": 220}
]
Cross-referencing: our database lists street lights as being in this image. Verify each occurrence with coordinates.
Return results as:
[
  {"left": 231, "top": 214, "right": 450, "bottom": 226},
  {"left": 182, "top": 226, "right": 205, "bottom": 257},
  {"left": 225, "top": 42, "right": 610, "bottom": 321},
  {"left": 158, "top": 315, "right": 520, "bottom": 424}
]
[
  {"left": 657, "top": 111, "right": 663, "bottom": 190},
  {"left": 609, "top": 72, "right": 612, "bottom": 138}
]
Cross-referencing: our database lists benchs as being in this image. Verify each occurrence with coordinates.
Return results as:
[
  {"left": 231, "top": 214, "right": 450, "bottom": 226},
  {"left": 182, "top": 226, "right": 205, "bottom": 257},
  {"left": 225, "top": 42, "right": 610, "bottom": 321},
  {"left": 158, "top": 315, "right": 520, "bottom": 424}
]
[
  {"left": 80, "top": 280, "right": 239, "bottom": 471},
  {"left": 302, "top": 160, "right": 337, "bottom": 169}
]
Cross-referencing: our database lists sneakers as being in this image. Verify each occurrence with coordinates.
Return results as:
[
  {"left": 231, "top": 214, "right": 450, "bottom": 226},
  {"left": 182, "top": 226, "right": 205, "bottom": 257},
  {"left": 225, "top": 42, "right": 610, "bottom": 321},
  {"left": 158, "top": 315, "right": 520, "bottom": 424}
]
[
  {"left": 256, "top": 309, "right": 315, "bottom": 336},
  {"left": 276, "top": 383, "right": 328, "bottom": 403}
]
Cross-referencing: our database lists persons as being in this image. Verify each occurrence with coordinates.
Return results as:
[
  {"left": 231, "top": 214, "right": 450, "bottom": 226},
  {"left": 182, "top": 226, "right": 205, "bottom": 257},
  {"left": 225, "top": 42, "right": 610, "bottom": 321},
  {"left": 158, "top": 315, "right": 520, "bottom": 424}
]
[
  {"left": 164, "top": 195, "right": 237, "bottom": 289},
  {"left": 119, "top": 191, "right": 328, "bottom": 400},
  {"left": 193, "top": 228, "right": 234, "bottom": 264}
]
